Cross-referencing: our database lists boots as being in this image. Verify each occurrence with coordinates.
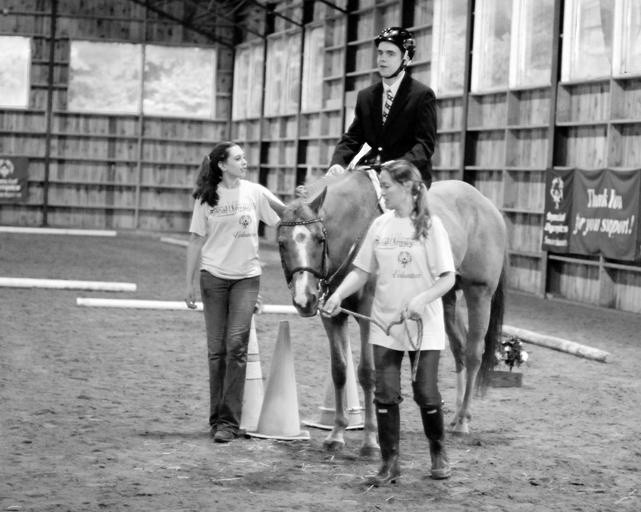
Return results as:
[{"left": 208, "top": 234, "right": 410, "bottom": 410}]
[
  {"left": 369, "top": 403, "right": 400, "bottom": 485},
  {"left": 421, "top": 405, "right": 451, "bottom": 479}
]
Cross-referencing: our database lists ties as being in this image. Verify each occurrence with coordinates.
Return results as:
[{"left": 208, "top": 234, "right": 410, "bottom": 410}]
[{"left": 382, "top": 91, "right": 393, "bottom": 126}]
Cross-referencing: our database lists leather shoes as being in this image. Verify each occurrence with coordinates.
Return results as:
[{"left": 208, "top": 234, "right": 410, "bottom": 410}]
[{"left": 214, "top": 430, "right": 233, "bottom": 442}]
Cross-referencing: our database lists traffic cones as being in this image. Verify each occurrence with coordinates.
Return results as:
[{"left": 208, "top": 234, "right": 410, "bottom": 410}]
[
  {"left": 247, "top": 318, "right": 313, "bottom": 445},
  {"left": 238, "top": 310, "right": 267, "bottom": 434}
]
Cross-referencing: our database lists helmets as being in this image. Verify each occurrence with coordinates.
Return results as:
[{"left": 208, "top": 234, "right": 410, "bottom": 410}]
[{"left": 375, "top": 27, "right": 416, "bottom": 57}]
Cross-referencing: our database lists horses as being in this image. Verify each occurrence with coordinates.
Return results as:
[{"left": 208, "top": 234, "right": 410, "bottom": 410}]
[{"left": 262, "top": 167, "right": 516, "bottom": 462}]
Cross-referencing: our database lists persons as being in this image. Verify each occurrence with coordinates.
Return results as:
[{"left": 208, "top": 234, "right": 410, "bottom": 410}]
[
  {"left": 326, "top": 26, "right": 438, "bottom": 190},
  {"left": 183, "top": 141, "right": 290, "bottom": 443},
  {"left": 321, "top": 158, "right": 457, "bottom": 488}
]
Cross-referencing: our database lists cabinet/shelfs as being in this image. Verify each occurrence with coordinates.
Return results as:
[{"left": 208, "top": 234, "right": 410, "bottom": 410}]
[{"left": 0, "top": 0, "right": 641, "bottom": 314}]
